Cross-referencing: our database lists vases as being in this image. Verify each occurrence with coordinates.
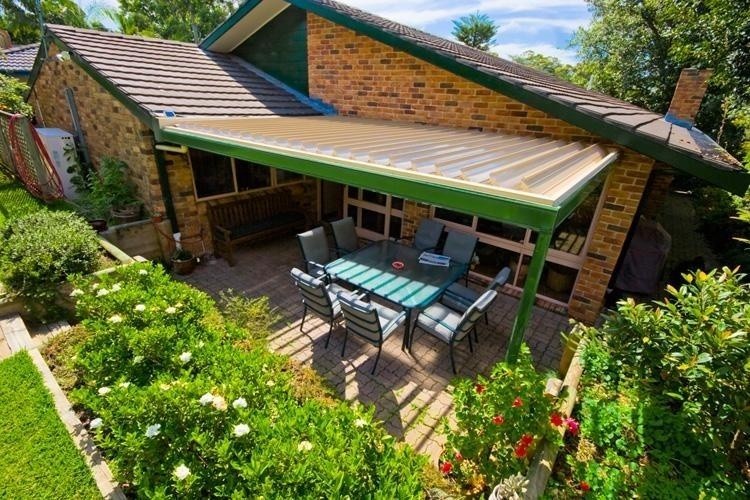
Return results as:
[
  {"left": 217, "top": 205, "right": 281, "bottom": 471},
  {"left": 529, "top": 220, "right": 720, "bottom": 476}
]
[
  {"left": 88, "top": 220, "right": 107, "bottom": 230},
  {"left": 438, "top": 449, "right": 462, "bottom": 474}
]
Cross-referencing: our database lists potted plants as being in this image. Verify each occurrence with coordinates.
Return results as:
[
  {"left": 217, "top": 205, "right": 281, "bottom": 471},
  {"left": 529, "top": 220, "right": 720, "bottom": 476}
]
[
  {"left": 87, "top": 149, "right": 143, "bottom": 223},
  {"left": 450, "top": 458, "right": 486, "bottom": 496},
  {"left": 151, "top": 210, "right": 161, "bottom": 223},
  {"left": 488, "top": 471, "right": 530, "bottom": 500},
  {"left": 170, "top": 247, "right": 193, "bottom": 275}
]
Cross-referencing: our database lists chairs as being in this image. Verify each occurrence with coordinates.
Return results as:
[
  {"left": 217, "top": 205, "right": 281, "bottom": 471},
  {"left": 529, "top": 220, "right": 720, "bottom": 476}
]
[{"left": 289, "top": 217, "right": 511, "bottom": 375}]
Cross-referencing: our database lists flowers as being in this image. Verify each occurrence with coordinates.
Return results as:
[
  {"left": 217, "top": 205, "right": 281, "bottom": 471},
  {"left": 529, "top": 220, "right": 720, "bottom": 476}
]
[{"left": 439, "top": 449, "right": 463, "bottom": 476}]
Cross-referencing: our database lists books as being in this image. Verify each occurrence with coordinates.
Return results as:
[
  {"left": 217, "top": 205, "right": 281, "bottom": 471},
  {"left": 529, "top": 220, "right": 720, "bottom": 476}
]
[{"left": 417, "top": 250, "right": 452, "bottom": 268}]
